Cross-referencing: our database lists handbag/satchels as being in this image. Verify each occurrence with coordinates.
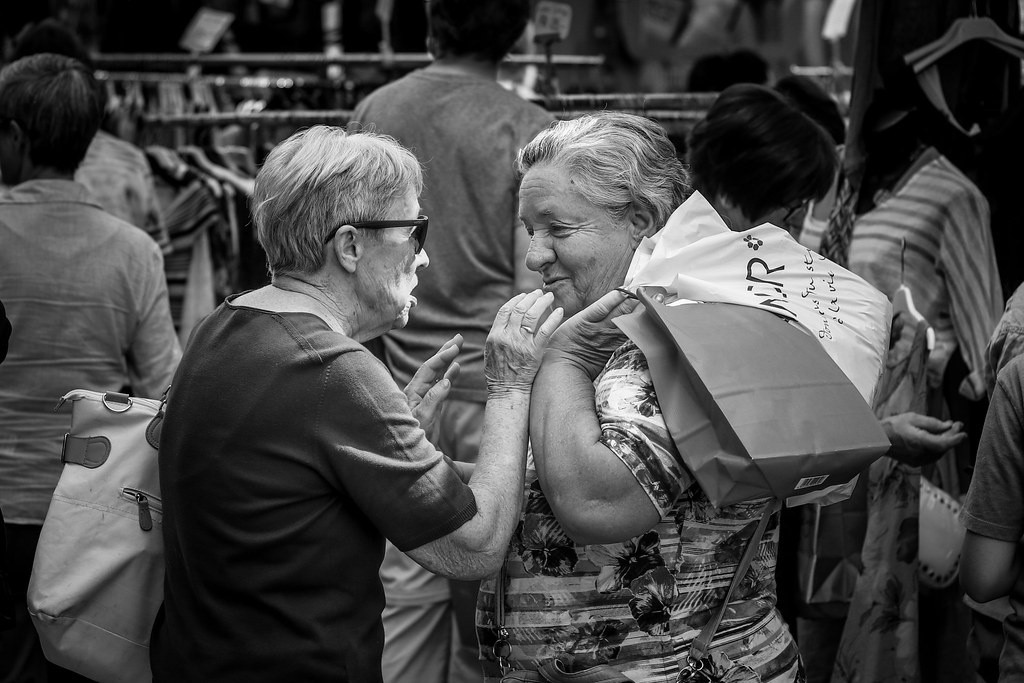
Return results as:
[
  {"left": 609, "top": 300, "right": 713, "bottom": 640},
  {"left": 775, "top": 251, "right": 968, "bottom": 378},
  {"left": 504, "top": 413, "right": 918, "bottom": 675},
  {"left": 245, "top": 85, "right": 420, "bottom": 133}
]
[
  {"left": 603, "top": 288, "right": 892, "bottom": 512},
  {"left": 618, "top": 186, "right": 895, "bottom": 511},
  {"left": 28, "top": 386, "right": 177, "bottom": 683},
  {"left": 908, "top": 477, "right": 968, "bottom": 588}
]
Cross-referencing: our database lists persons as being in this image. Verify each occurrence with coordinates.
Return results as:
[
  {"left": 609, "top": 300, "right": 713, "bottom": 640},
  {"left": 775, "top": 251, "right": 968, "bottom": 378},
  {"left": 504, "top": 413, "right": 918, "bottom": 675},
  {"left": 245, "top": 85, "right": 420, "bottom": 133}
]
[
  {"left": 0, "top": 19, "right": 183, "bottom": 683},
  {"left": 149, "top": 125, "right": 563, "bottom": 683},
  {"left": 435, "top": 112, "right": 808, "bottom": 683},
  {"left": 348, "top": 0, "right": 559, "bottom": 683},
  {"left": 687, "top": 47, "right": 1024, "bottom": 683}
]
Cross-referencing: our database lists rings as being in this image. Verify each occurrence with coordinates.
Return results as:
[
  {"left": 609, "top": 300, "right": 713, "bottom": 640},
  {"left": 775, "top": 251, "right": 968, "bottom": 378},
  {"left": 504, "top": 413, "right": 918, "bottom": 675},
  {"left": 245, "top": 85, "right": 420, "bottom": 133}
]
[{"left": 522, "top": 325, "right": 534, "bottom": 334}]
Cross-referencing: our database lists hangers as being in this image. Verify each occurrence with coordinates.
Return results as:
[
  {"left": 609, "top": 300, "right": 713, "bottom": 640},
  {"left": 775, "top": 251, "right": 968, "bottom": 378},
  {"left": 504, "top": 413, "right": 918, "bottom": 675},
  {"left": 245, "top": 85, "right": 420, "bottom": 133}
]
[
  {"left": 540, "top": 60, "right": 854, "bottom": 146},
  {"left": 91, "top": 65, "right": 368, "bottom": 177}
]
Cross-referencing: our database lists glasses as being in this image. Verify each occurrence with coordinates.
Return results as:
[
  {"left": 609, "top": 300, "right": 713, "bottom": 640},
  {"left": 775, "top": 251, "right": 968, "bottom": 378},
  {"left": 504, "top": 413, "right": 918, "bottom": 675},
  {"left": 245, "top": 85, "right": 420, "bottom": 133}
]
[{"left": 320, "top": 213, "right": 429, "bottom": 253}]
